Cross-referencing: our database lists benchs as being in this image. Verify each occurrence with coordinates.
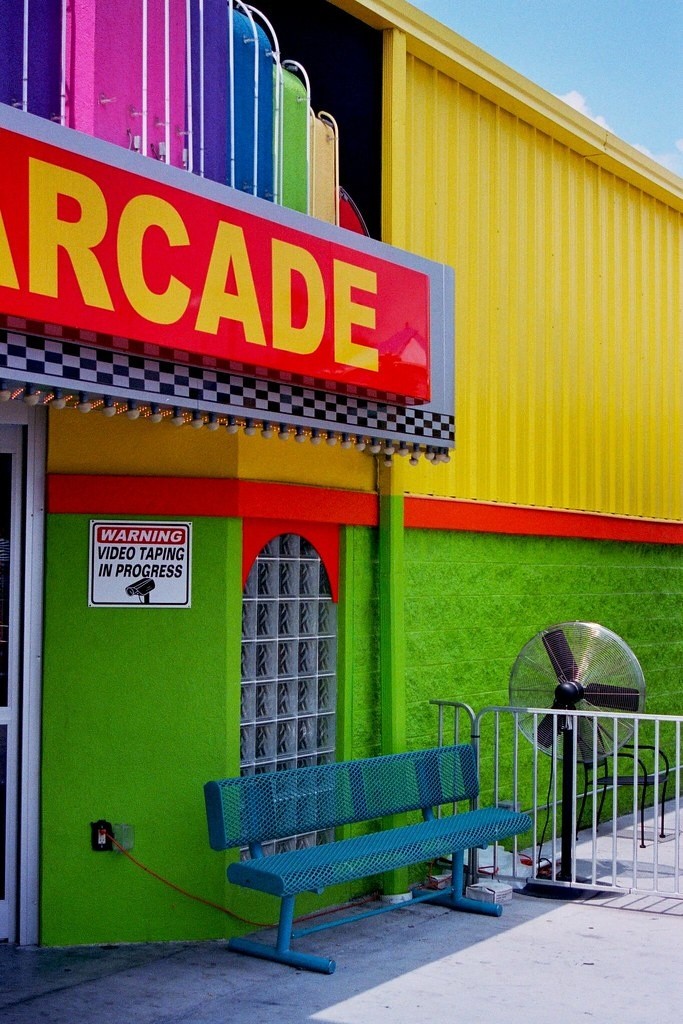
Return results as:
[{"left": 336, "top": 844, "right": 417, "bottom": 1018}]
[{"left": 203, "top": 743, "right": 533, "bottom": 975}]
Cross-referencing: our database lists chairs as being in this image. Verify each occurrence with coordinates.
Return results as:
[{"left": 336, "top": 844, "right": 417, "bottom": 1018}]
[{"left": 569, "top": 716, "right": 670, "bottom": 848}]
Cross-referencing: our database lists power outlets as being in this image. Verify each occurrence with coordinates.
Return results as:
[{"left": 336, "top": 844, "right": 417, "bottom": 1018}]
[{"left": 91, "top": 821, "right": 114, "bottom": 852}]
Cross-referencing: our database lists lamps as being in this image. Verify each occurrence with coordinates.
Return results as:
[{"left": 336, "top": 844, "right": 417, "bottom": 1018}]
[{"left": 0, "top": 379, "right": 452, "bottom": 468}]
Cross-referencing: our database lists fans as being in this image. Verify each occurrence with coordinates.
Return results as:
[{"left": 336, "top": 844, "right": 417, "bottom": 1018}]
[{"left": 509, "top": 620, "right": 647, "bottom": 902}]
